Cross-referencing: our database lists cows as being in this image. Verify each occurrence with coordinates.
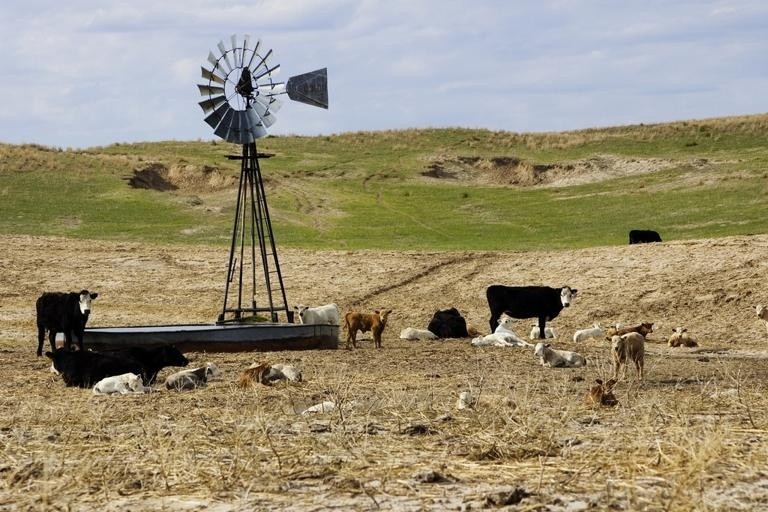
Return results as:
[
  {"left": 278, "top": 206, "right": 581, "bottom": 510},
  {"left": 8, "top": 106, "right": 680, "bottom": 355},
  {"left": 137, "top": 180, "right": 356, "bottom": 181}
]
[
  {"left": 486, "top": 286, "right": 577, "bottom": 338},
  {"left": 45, "top": 342, "right": 222, "bottom": 396},
  {"left": 301, "top": 401, "right": 365, "bottom": 416},
  {"left": 530, "top": 326, "right": 557, "bottom": 340},
  {"left": 668, "top": 327, "right": 699, "bottom": 347},
  {"left": 629, "top": 230, "right": 663, "bottom": 244},
  {"left": 36, "top": 290, "right": 98, "bottom": 361},
  {"left": 244, "top": 359, "right": 302, "bottom": 387},
  {"left": 471, "top": 317, "right": 535, "bottom": 347},
  {"left": 526, "top": 343, "right": 586, "bottom": 368},
  {"left": 572, "top": 322, "right": 654, "bottom": 343},
  {"left": 343, "top": 306, "right": 392, "bottom": 350},
  {"left": 428, "top": 307, "right": 469, "bottom": 338},
  {"left": 605, "top": 332, "right": 644, "bottom": 382},
  {"left": 752, "top": 304, "right": 768, "bottom": 333},
  {"left": 296, "top": 303, "right": 339, "bottom": 325},
  {"left": 400, "top": 327, "right": 439, "bottom": 341},
  {"left": 450, "top": 391, "right": 516, "bottom": 410},
  {"left": 589, "top": 377, "right": 624, "bottom": 411}
]
